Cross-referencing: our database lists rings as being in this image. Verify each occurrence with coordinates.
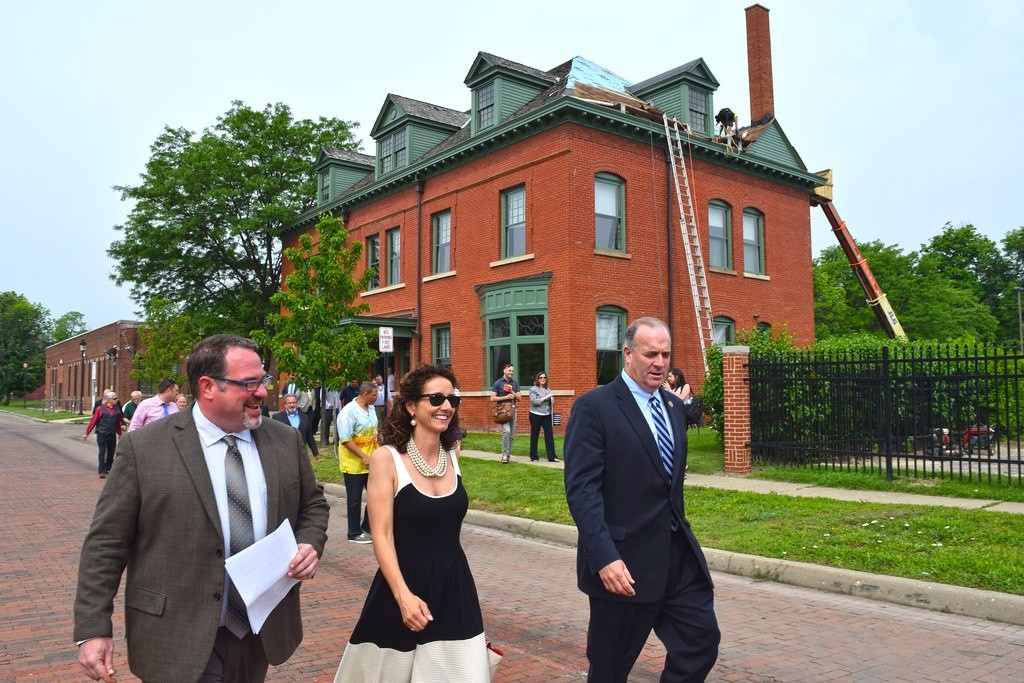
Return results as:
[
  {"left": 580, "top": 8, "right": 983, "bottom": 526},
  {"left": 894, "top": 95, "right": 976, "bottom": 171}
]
[{"left": 411, "top": 626, "right": 416, "bottom": 632}]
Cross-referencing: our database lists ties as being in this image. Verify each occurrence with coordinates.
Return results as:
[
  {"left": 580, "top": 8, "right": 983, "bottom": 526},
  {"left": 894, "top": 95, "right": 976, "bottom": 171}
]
[
  {"left": 163, "top": 403, "right": 168, "bottom": 417},
  {"left": 220, "top": 436, "right": 257, "bottom": 640},
  {"left": 647, "top": 395, "right": 675, "bottom": 483}
]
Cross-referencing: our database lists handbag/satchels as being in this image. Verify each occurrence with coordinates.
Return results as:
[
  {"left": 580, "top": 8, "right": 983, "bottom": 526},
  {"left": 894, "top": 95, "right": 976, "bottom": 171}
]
[
  {"left": 685, "top": 397, "right": 705, "bottom": 424},
  {"left": 492, "top": 403, "right": 513, "bottom": 424}
]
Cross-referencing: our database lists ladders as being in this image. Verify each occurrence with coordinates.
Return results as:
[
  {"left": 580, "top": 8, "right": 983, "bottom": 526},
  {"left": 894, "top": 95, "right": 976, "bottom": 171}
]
[{"left": 661, "top": 112, "right": 717, "bottom": 427}]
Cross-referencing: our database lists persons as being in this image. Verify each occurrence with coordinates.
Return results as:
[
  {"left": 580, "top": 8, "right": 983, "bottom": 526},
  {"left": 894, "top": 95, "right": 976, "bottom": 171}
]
[
  {"left": 490, "top": 364, "right": 521, "bottom": 463},
  {"left": 252, "top": 366, "right": 397, "bottom": 461},
  {"left": 335, "top": 381, "right": 384, "bottom": 544},
  {"left": 331, "top": 362, "right": 491, "bottom": 683},
  {"left": 661, "top": 368, "right": 691, "bottom": 479},
  {"left": 82, "top": 378, "right": 187, "bottom": 478},
  {"left": 563, "top": 316, "right": 721, "bottom": 683},
  {"left": 528, "top": 372, "right": 560, "bottom": 462},
  {"left": 71, "top": 336, "right": 331, "bottom": 683}
]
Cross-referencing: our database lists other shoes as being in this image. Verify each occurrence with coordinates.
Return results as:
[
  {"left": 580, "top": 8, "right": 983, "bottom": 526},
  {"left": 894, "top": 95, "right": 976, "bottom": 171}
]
[
  {"left": 549, "top": 457, "right": 559, "bottom": 462},
  {"left": 501, "top": 456, "right": 510, "bottom": 463},
  {"left": 99, "top": 473, "right": 106, "bottom": 478},
  {"left": 361, "top": 529, "right": 373, "bottom": 539},
  {"left": 532, "top": 458, "right": 539, "bottom": 462},
  {"left": 348, "top": 535, "right": 373, "bottom": 544}
]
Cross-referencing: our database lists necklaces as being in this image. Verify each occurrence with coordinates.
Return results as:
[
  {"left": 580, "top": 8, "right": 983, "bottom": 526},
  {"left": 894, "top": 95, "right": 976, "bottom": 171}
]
[{"left": 405, "top": 432, "right": 448, "bottom": 477}]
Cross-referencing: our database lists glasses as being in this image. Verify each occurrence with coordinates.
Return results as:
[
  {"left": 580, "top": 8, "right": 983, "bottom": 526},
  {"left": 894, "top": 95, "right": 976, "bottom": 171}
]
[
  {"left": 285, "top": 402, "right": 298, "bottom": 405},
  {"left": 206, "top": 374, "right": 273, "bottom": 392},
  {"left": 414, "top": 393, "right": 461, "bottom": 408},
  {"left": 539, "top": 377, "right": 546, "bottom": 379},
  {"left": 110, "top": 397, "right": 118, "bottom": 401}
]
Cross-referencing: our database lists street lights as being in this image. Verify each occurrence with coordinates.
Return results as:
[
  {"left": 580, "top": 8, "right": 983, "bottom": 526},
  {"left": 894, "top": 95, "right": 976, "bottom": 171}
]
[
  {"left": 79, "top": 339, "right": 86, "bottom": 416},
  {"left": 24, "top": 363, "right": 27, "bottom": 409}
]
